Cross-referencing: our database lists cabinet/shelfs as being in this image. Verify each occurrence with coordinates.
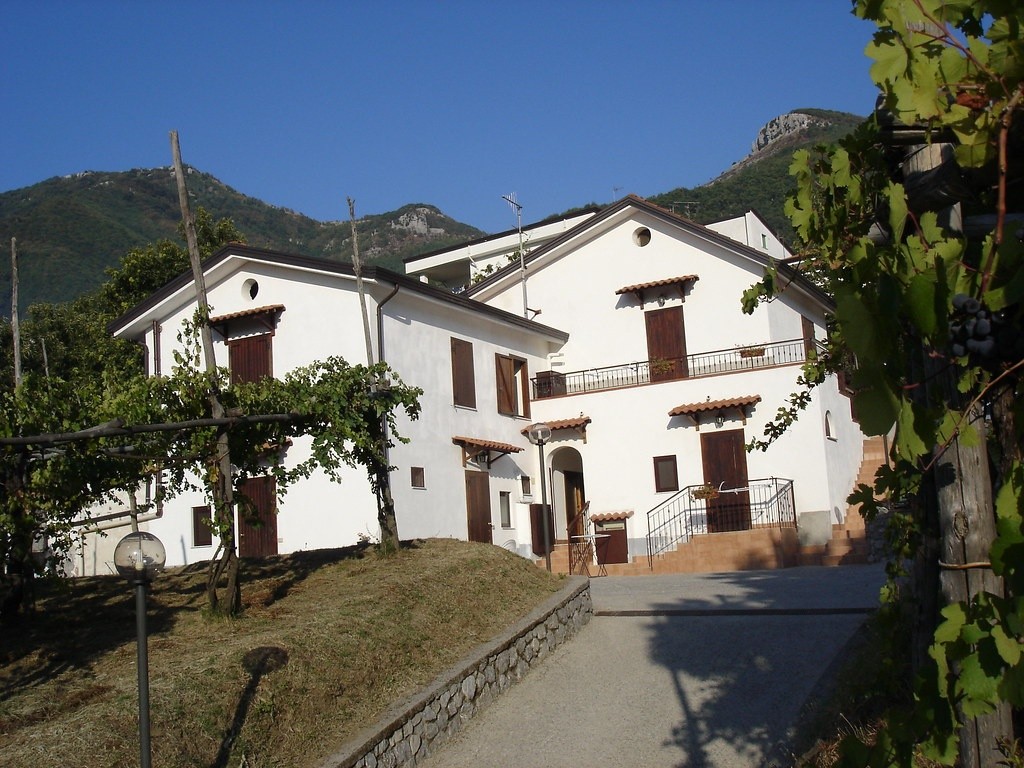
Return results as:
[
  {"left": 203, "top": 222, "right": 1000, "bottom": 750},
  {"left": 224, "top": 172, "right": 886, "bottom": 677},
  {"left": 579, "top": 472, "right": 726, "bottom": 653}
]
[{"left": 536, "top": 371, "right": 567, "bottom": 398}]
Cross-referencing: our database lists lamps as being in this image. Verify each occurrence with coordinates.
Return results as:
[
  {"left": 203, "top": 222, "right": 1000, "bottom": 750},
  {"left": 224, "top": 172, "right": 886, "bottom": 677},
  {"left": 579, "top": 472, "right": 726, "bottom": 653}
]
[
  {"left": 715, "top": 410, "right": 725, "bottom": 427},
  {"left": 658, "top": 293, "right": 666, "bottom": 305},
  {"left": 113, "top": 532, "right": 166, "bottom": 768},
  {"left": 476, "top": 452, "right": 489, "bottom": 463}
]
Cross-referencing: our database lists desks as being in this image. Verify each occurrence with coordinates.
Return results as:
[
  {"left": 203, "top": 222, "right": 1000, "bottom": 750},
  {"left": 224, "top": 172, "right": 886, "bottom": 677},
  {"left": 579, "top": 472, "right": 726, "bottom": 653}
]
[{"left": 570, "top": 532, "right": 611, "bottom": 577}]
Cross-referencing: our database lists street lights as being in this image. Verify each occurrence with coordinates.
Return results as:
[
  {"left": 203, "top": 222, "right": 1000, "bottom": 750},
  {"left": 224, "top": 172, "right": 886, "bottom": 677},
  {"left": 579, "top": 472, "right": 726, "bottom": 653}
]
[{"left": 528, "top": 422, "right": 551, "bottom": 574}]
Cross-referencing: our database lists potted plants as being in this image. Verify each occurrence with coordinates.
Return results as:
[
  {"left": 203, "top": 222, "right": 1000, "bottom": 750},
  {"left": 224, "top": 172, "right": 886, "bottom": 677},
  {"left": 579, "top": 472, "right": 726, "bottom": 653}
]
[
  {"left": 734, "top": 341, "right": 768, "bottom": 358},
  {"left": 648, "top": 355, "right": 677, "bottom": 376},
  {"left": 691, "top": 481, "right": 721, "bottom": 502}
]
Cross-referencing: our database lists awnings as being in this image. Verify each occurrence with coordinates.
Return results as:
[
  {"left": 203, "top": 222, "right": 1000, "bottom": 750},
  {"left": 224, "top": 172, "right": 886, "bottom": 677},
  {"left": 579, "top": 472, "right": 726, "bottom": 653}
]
[
  {"left": 521, "top": 417, "right": 591, "bottom": 440},
  {"left": 208, "top": 304, "right": 284, "bottom": 338},
  {"left": 615, "top": 275, "right": 699, "bottom": 299},
  {"left": 668, "top": 395, "right": 760, "bottom": 425},
  {"left": 453, "top": 436, "right": 523, "bottom": 464}
]
